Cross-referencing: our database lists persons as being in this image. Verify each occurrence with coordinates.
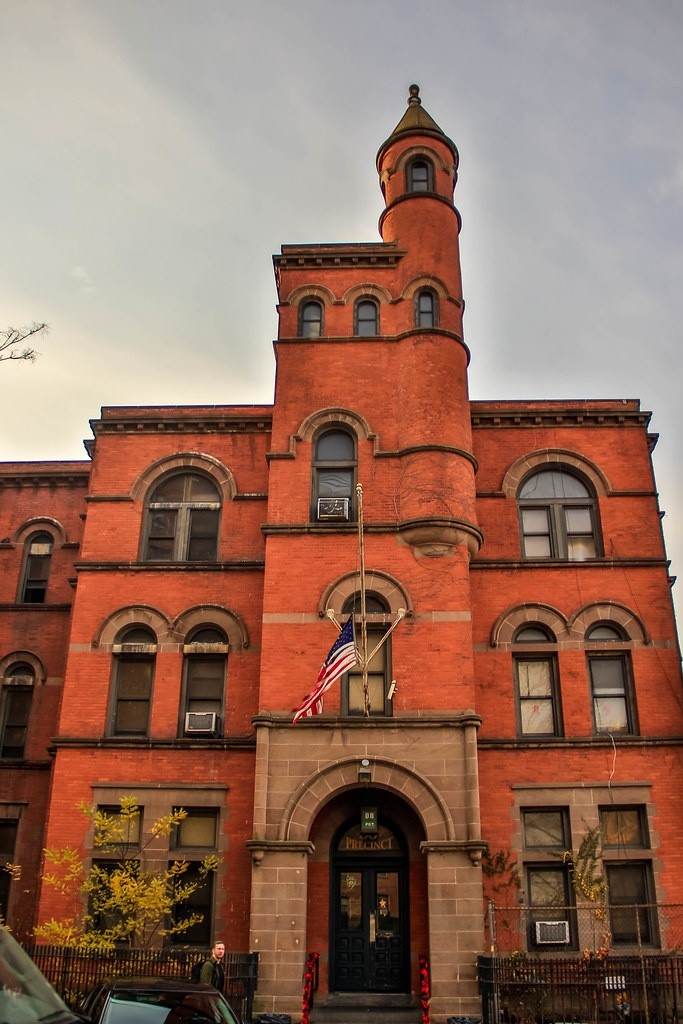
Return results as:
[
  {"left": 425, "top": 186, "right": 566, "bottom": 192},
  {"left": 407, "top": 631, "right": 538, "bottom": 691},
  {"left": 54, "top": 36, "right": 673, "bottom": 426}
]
[{"left": 197, "top": 941, "right": 227, "bottom": 998}]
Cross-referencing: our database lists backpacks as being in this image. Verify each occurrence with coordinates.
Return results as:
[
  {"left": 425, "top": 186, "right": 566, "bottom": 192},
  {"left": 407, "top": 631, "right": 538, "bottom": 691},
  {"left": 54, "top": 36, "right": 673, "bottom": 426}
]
[{"left": 191, "top": 957, "right": 215, "bottom": 985}]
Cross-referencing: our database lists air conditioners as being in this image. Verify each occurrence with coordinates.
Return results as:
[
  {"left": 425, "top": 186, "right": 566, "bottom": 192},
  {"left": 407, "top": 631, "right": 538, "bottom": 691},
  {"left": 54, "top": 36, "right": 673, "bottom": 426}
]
[
  {"left": 535, "top": 921, "right": 570, "bottom": 943},
  {"left": 184, "top": 712, "right": 217, "bottom": 734},
  {"left": 317, "top": 497, "right": 349, "bottom": 521}
]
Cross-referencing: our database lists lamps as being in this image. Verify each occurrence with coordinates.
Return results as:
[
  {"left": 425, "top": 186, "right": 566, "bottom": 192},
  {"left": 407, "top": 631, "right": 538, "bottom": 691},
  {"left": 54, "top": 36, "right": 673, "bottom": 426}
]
[{"left": 358, "top": 767, "right": 371, "bottom": 783}]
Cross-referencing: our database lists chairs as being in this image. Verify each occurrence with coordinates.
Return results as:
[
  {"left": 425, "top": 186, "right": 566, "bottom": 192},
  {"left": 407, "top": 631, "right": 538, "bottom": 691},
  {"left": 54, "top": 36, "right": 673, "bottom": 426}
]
[{"left": 114, "top": 995, "right": 203, "bottom": 1021}]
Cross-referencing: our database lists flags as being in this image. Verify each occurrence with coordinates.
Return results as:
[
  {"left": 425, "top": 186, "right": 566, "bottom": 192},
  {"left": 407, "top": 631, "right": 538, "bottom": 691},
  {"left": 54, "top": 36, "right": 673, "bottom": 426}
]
[{"left": 289, "top": 608, "right": 364, "bottom": 729}]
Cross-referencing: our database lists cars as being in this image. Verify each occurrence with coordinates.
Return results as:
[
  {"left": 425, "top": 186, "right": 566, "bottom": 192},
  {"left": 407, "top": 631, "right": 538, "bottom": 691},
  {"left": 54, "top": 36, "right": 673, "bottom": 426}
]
[{"left": 73, "top": 973, "right": 245, "bottom": 1024}]
